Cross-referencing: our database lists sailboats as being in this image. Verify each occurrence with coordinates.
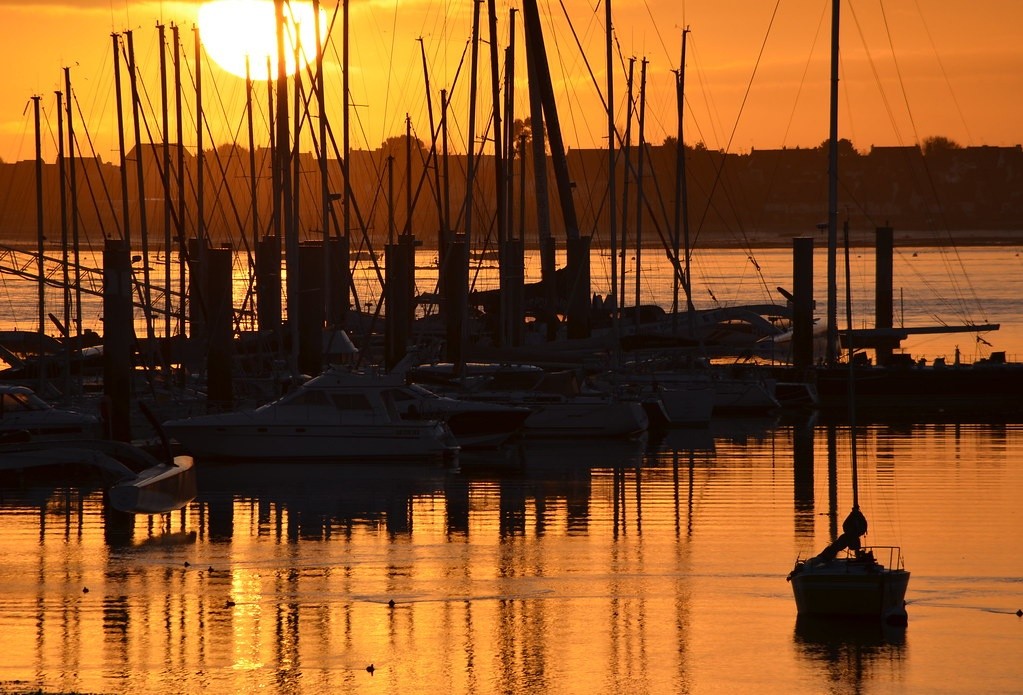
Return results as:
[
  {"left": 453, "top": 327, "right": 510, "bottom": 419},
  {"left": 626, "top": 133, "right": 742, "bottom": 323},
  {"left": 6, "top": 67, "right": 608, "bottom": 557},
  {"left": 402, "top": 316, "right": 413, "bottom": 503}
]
[
  {"left": 786, "top": 219, "right": 911, "bottom": 625},
  {"left": 0, "top": 1, "right": 1002, "bottom": 468}
]
